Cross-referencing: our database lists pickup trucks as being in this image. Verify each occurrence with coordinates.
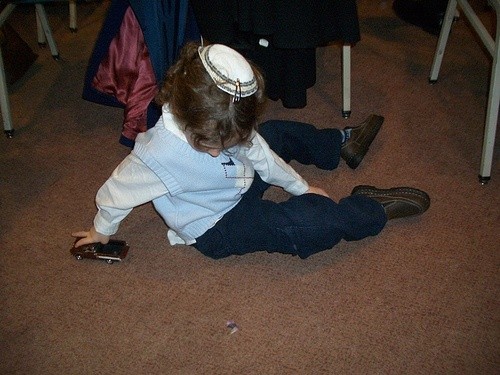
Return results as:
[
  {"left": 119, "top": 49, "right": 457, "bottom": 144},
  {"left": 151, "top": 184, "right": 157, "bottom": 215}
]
[{"left": 70, "top": 237, "right": 131, "bottom": 264}]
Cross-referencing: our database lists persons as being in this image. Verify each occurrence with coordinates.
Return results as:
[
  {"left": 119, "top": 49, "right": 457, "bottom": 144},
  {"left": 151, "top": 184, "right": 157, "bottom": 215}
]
[
  {"left": 72, "top": 43, "right": 430, "bottom": 260},
  {"left": 0, "top": 0, "right": 454, "bottom": 145}
]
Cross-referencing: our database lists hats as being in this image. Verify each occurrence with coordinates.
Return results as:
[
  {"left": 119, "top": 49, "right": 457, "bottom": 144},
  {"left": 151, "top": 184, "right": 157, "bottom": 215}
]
[{"left": 197, "top": 43, "right": 259, "bottom": 103}]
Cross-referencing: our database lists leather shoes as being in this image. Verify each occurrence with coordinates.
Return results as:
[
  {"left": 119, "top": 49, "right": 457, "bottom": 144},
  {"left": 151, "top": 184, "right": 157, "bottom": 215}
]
[
  {"left": 337, "top": 113, "right": 385, "bottom": 170},
  {"left": 350, "top": 184, "right": 431, "bottom": 220}
]
